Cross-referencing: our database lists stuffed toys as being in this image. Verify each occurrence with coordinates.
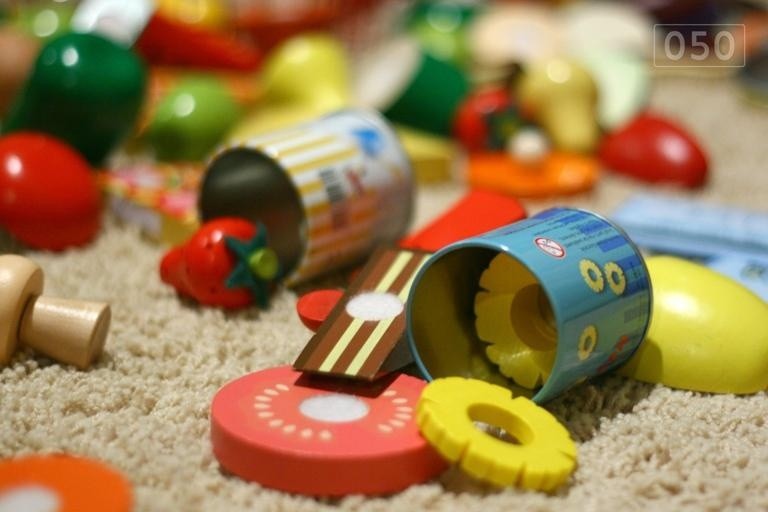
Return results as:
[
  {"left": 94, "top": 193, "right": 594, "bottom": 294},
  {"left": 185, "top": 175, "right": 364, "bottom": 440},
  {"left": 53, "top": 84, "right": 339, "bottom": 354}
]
[{"left": 0, "top": 1, "right": 766, "bottom": 510}]
